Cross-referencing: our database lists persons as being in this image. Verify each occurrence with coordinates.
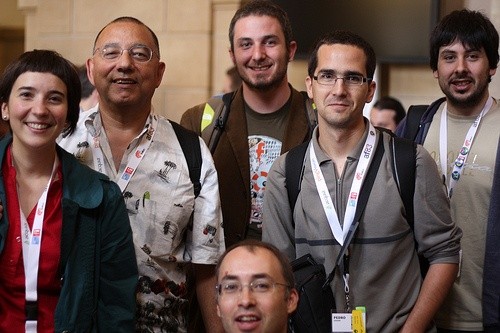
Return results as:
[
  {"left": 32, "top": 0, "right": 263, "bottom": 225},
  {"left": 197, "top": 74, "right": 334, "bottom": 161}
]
[{"left": 0, "top": 1, "right": 498, "bottom": 333}]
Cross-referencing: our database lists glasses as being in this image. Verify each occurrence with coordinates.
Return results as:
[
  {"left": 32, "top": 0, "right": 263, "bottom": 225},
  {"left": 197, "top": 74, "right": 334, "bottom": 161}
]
[
  {"left": 211, "top": 279, "right": 289, "bottom": 296},
  {"left": 92, "top": 44, "right": 159, "bottom": 64},
  {"left": 314, "top": 69, "right": 373, "bottom": 87}
]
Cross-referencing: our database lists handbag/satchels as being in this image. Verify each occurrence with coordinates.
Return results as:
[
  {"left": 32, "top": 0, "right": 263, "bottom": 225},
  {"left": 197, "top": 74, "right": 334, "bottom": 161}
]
[{"left": 290, "top": 264, "right": 337, "bottom": 333}]
